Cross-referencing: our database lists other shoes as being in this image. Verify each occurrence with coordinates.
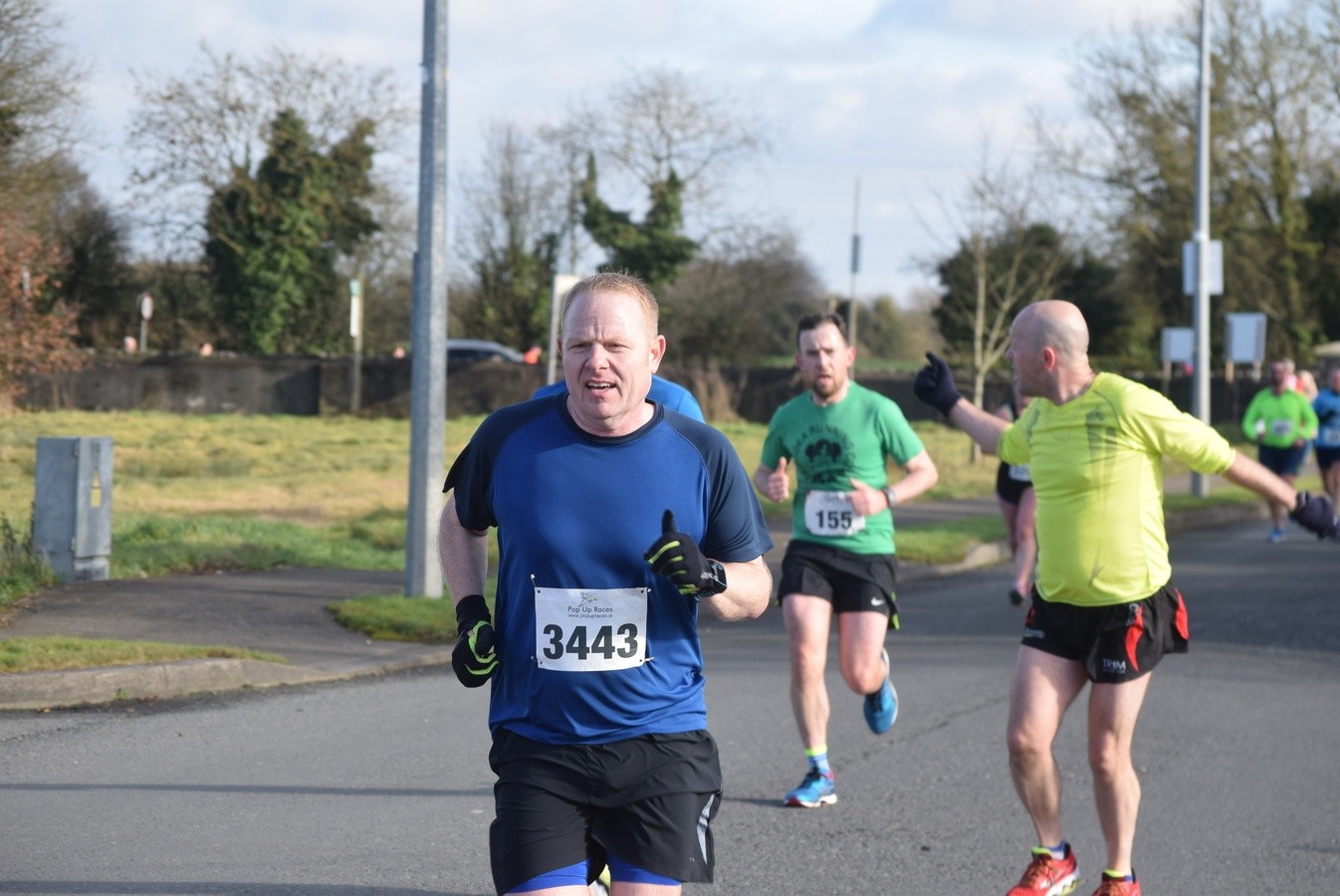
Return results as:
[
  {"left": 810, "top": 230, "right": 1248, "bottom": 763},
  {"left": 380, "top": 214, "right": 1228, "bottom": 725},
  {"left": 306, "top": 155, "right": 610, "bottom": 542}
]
[
  {"left": 1267, "top": 525, "right": 1288, "bottom": 543},
  {"left": 1010, "top": 584, "right": 1026, "bottom": 606}
]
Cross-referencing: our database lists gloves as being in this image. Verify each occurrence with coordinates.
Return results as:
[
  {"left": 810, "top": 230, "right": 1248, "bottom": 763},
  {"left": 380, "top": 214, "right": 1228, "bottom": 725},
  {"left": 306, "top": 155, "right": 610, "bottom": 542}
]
[
  {"left": 450, "top": 620, "right": 500, "bottom": 690},
  {"left": 1289, "top": 492, "right": 1340, "bottom": 540},
  {"left": 644, "top": 508, "right": 711, "bottom": 595},
  {"left": 913, "top": 350, "right": 965, "bottom": 417}
]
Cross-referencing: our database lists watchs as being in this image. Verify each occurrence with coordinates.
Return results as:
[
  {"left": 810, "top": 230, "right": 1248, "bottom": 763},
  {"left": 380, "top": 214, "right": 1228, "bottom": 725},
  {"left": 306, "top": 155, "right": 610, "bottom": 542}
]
[{"left": 698, "top": 559, "right": 727, "bottom": 597}]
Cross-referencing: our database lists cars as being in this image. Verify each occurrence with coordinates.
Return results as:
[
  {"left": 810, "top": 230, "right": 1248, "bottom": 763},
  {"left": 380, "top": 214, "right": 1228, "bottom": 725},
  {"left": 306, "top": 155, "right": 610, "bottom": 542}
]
[{"left": 446, "top": 339, "right": 527, "bottom": 365}]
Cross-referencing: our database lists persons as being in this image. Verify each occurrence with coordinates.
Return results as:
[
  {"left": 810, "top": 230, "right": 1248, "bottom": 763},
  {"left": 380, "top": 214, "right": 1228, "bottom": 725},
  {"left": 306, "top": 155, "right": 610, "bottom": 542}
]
[
  {"left": 436, "top": 269, "right": 775, "bottom": 896},
  {"left": 529, "top": 374, "right": 707, "bottom": 896},
  {"left": 752, "top": 313, "right": 938, "bottom": 808},
  {"left": 915, "top": 299, "right": 1340, "bottom": 896},
  {"left": 1242, "top": 358, "right": 1340, "bottom": 545},
  {"left": 981, "top": 376, "right": 1037, "bottom": 607}
]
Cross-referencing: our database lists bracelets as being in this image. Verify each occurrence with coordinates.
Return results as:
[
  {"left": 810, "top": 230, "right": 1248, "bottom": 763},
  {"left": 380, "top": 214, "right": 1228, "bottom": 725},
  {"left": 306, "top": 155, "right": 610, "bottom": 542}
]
[{"left": 882, "top": 486, "right": 896, "bottom": 509}]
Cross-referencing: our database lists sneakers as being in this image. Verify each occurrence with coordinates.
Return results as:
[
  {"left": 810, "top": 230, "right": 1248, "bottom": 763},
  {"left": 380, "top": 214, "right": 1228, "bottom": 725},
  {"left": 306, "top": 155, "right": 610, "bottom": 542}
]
[
  {"left": 863, "top": 647, "right": 898, "bottom": 735},
  {"left": 1091, "top": 868, "right": 1141, "bottom": 896},
  {"left": 782, "top": 765, "right": 838, "bottom": 807},
  {"left": 1005, "top": 842, "right": 1080, "bottom": 896}
]
